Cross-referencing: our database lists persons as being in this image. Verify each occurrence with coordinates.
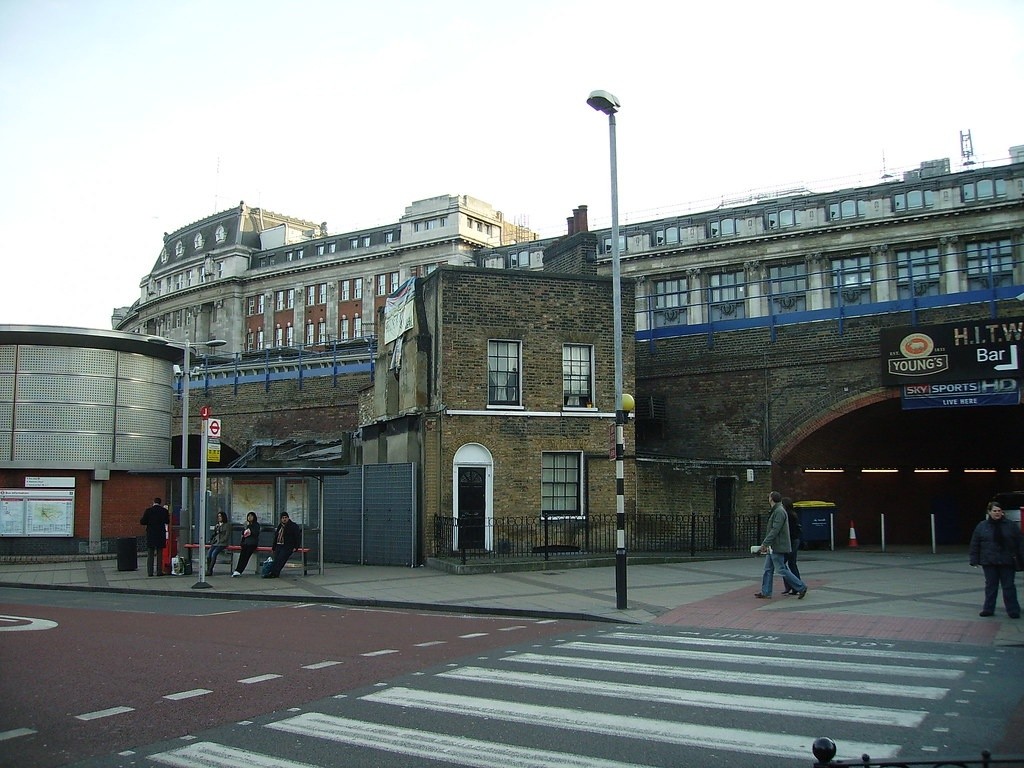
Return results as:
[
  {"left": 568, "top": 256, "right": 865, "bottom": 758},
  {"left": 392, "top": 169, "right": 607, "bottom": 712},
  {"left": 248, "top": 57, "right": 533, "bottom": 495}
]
[
  {"left": 262, "top": 512, "right": 300, "bottom": 578},
  {"left": 140, "top": 498, "right": 170, "bottom": 577},
  {"left": 969, "top": 501, "right": 1024, "bottom": 618},
  {"left": 231, "top": 512, "right": 260, "bottom": 578},
  {"left": 205, "top": 512, "right": 231, "bottom": 576},
  {"left": 755, "top": 491, "right": 807, "bottom": 598}
]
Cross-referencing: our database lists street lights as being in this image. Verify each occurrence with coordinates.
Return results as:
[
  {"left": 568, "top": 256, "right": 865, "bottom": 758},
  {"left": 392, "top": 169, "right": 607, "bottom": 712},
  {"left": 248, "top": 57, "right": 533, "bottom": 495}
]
[
  {"left": 585, "top": 88, "right": 634, "bottom": 610},
  {"left": 145, "top": 335, "right": 228, "bottom": 574}
]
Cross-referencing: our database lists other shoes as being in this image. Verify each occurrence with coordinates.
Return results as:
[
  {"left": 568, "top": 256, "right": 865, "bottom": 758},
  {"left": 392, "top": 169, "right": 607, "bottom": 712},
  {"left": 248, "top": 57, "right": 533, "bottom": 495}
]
[
  {"left": 754, "top": 593, "right": 771, "bottom": 599},
  {"left": 798, "top": 586, "right": 808, "bottom": 599},
  {"left": 231, "top": 571, "right": 242, "bottom": 577},
  {"left": 148, "top": 572, "right": 153, "bottom": 576},
  {"left": 782, "top": 587, "right": 791, "bottom": 594},
  {"left": 789, "top": 589, "right": 798, "bottom": 595},
  {"left": 157, "top": 572, "right": 166, "bottom": 576},
  {"left": 1010, "top": 612, "right": 1021, "bottom": 618},
  {"left": 262, "top": 572, "right": 279, "bottom": 578},
  {"left": 980, "top": 611, "right": 994, "bottom": 616}
]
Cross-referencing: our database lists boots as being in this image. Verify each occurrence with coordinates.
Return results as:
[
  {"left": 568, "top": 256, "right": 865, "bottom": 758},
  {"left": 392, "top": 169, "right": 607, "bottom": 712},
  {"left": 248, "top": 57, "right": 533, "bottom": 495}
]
[{"left": 205, "top": 558, "right": 216, "bottom": 576}]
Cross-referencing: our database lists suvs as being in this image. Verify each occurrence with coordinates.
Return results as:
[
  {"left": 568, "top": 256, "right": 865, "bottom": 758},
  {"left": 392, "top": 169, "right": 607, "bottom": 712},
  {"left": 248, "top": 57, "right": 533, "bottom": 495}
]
[{"left": 982, "top": 490, "right": 1024, "bottom": 530}]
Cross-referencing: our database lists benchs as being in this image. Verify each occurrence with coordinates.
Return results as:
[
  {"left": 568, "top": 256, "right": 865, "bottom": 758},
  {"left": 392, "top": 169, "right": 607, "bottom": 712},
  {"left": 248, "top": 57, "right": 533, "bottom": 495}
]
[{"left": 184, "top": 543, "right": 311, "bottom": 576}]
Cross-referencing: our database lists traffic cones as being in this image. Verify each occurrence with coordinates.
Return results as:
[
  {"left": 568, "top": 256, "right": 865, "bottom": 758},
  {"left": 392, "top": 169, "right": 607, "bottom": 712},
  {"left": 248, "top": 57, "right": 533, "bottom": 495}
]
[{"left": 847, "top": 519, "right": 859, "bottom": 547}]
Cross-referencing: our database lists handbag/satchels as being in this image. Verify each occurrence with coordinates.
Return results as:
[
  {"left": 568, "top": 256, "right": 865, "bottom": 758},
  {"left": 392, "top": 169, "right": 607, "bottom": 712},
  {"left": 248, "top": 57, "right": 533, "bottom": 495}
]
[
  {"left": 261, "top": 557, "right": 274, "bottom": 575},
  {"left": 170, "top": 555, "right": 185, "bottom": 575},
  {"left": 184, "top": 560, "right": 193, "bottom": 575}
]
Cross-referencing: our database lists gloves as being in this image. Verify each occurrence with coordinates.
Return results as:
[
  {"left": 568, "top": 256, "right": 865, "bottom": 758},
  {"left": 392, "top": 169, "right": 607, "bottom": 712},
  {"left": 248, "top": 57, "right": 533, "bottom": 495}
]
[
  {"left": 243, "top": 528, "right": 252, "bottom": 537},
  {"left": 968, "top": 563, "right": 978, "bottom": 569}
]
